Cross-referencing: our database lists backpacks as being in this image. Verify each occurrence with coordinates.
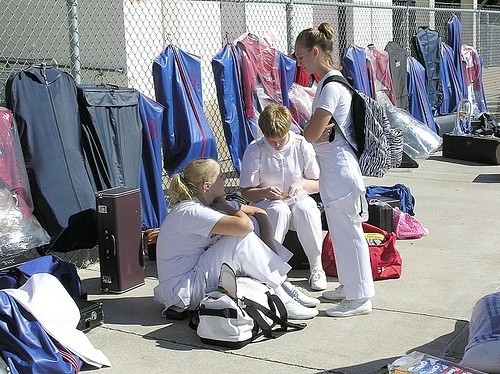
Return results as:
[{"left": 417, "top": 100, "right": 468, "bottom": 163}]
[{"left": 318, "top": 75, "right": 404, "bottom": 178}]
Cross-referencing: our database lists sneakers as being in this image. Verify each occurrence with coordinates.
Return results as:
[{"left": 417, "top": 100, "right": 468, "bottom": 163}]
[
  {"left": 322, "top": 284, "right": 346, "bottom": 300},
  {"left": 287, "top": 285, "right": 321, "bottom": 307},
  {"left": 309, "top": 266, "right": 327, "bottom": 290},
  {"left": 326, "top": 297, "right": 372, "bottom": 317},
  {"left": 284, "top": 297, "right": 319, "bottom": 319}
]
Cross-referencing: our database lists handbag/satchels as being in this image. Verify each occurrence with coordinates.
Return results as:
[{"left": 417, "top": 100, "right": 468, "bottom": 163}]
[
  {"left": 321, "top": 223, "right": 403, "bottom": 282},
  {"left": 364, "top": 183, "right": 415, "bottom": 216},
  {"left": 391, "top": 206, "right": 429, "bottom": 240},
  {"left": 0, "top": 254, "right": 87, "bottom": 301},
  {"left": 189, "top": 262, "right": 307, "bottom": 350}
]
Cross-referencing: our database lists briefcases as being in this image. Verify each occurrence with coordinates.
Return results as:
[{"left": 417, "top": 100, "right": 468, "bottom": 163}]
[
  {"left": 443, "top": 132, "right": 500, "bottom": 165},
  {"left": 378, "top": 196, "right": 401, "bottom": 210},
  {"left": 94, "top": 186, "right": 146, "bottom": 294},
  {"left": 72, "top": 297, "right": 104, "bottom": 333},
  {"left": 368, "top": 197, "right": 393, "bottom": 234}
]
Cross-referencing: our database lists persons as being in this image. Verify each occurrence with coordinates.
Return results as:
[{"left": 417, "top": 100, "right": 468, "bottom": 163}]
[
  {"left": 295, "top": 22, "right": 375, "bottom": 318},
  {"left": 155, "top": 158, "right": 320, "bottom": 320},
  {"left": 241, "top": 104, "right": 328, "bottom": 290}
]
